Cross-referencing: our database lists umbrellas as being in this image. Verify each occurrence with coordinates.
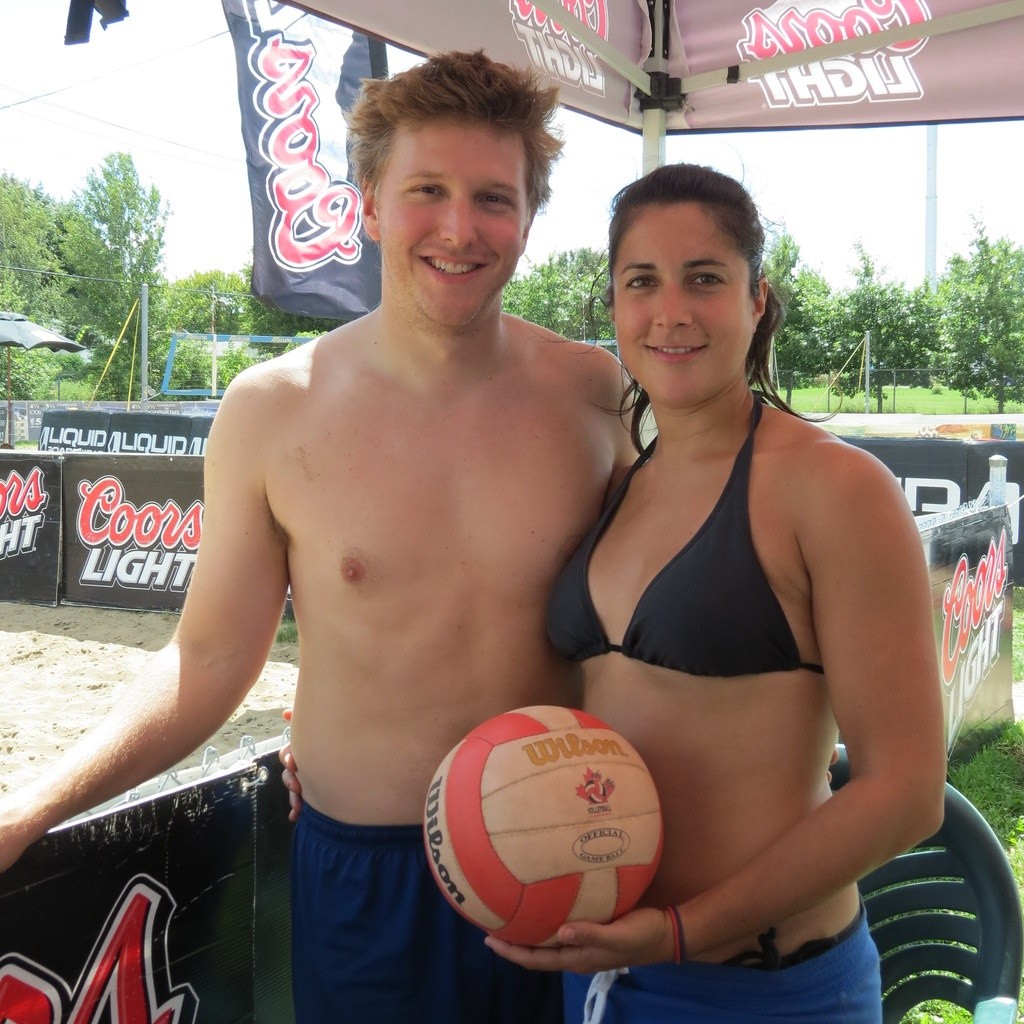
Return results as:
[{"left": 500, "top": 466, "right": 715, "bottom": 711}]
[{"left": 0, "top": 310, "right": 87, "bottom": 443}]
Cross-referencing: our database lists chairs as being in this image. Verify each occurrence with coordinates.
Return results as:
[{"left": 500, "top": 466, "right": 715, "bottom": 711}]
[{"left": 830, "top": 744, "right": 1023, "bottom": 1024}]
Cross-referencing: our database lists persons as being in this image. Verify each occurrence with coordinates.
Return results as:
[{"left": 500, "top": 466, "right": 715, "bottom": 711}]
[
  {"left": 0, "top": 53, "right": 635, "bottom": 1023},
  {"left": 279, "top": 164, "right": 944, "bottom": 1024}
]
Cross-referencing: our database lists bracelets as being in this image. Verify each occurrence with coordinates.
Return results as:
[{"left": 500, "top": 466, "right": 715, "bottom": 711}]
[{"left": 668, "top": 905, "right": 688, "bottom": 964}]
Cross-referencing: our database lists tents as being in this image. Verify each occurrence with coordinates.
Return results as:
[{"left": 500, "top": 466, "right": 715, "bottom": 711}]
[{"left": 277, "top": 0, "right": 1023, "bottom": 179}]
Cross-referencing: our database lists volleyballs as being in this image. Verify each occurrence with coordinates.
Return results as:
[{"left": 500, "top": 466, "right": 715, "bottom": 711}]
[{"left": 423, "top": 705, "right": 664, "bottom": 948}]
